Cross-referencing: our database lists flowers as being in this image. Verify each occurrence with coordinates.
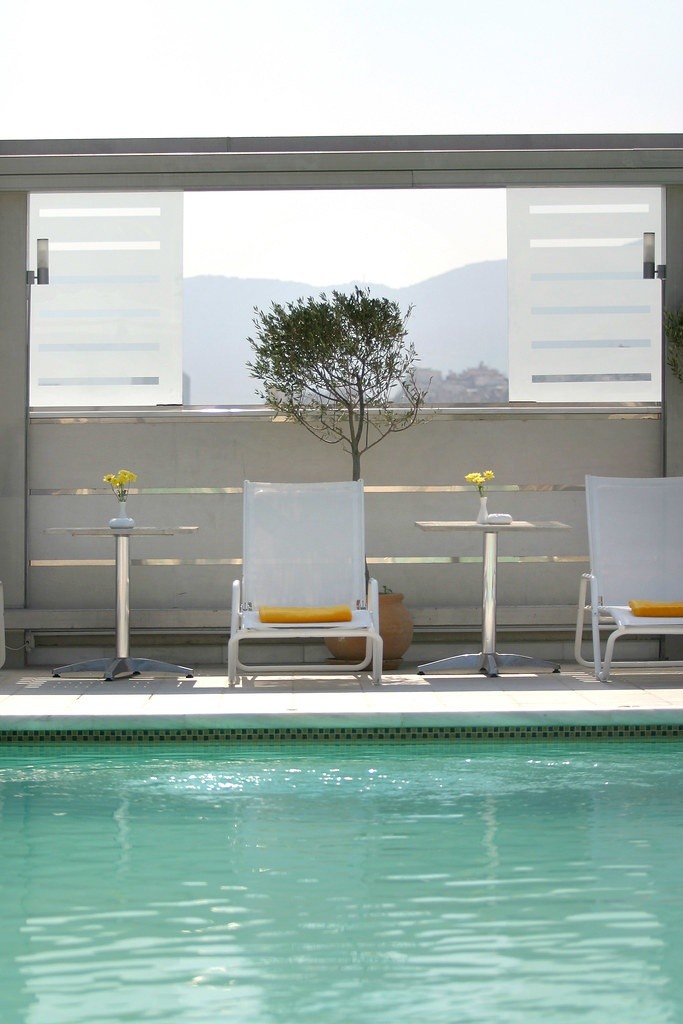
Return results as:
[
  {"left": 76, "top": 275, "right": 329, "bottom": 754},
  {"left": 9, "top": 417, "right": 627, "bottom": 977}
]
[
  {"left": 103, "top": 470, "right": 137, "bottom": 502},
  {"left": 465, "top": 470, "right": 495, "bottom": 496}
]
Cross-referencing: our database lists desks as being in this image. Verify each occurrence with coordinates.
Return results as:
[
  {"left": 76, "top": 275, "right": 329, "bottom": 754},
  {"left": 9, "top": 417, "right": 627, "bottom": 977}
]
[
  {"left": 412, "top": 520, "right": 573, "bottom": 678},
  {"left": 45, "top": 527, "right": 200, "bottom": 680}
]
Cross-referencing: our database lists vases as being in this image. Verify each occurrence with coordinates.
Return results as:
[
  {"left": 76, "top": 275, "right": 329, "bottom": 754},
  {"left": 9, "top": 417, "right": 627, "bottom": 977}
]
[
  {"left": 476, "top": 497, "right": 489, "bottom": 524},
  {"left": 109, "top": 502, "right": 135, "bottom": 528}
]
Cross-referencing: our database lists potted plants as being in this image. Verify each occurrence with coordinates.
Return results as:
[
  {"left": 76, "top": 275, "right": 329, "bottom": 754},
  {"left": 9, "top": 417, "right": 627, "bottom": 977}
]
[{"left": 247, "top": 288, "right": 415, "bottom": 669}]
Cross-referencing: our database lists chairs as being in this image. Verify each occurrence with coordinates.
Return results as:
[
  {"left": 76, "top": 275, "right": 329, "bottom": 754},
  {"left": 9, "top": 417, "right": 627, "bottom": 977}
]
[
  {"left": 575, "top": 474, "right": 683, "bottom": 682},
  {"left": 227, "top": 479, "right": 385, "bottom": 685}
]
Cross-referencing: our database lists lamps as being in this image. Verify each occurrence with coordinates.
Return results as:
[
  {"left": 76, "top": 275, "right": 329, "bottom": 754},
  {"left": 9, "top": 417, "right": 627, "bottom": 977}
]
[
  {"left": 643, "top": 232, "right": 666, "bottom": 279},
  {"left": 25, "top": 237, "right": 48, "bottom": 283}
]
